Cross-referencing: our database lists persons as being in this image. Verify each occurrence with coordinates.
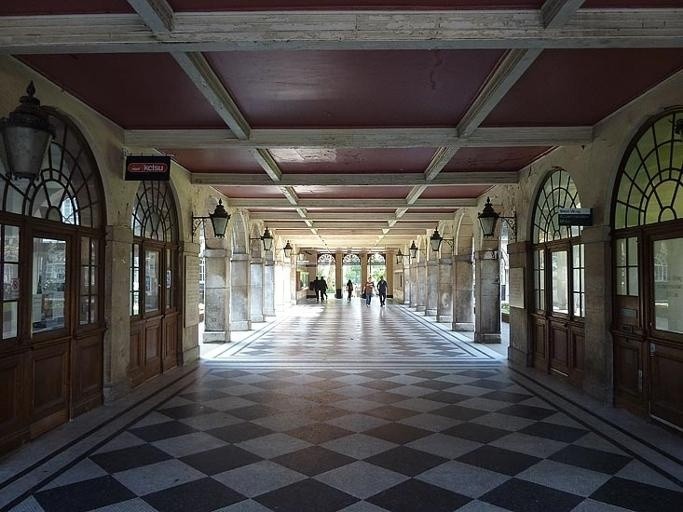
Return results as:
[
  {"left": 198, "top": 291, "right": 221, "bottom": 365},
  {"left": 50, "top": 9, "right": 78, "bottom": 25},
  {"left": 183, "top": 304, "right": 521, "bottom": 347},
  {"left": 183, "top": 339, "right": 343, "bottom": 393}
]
[
  {"left": 313, "top": 276, "right": 328, "bottom": 302},
  {"left": 347, "top": 280, "right": 353, "bottom": 303},
  {"left": 363, "top": 277, "right": 374, "bottom": 307},
  {"left": 377, "top": 276, "right": 388, "bottom": 307}
]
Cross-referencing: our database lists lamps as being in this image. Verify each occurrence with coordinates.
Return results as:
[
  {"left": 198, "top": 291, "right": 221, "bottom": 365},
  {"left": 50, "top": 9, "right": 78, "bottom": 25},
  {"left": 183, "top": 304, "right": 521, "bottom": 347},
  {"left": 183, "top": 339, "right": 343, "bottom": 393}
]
[
  {"left": 409, "top": 241, "right": 427, "bottom": 260},
  {"left": 248, "top": 226, "right": 273, "bottom": 251},
  {"left": 192, "top": 198, "right": 231, "bottom": 242},
  {"left": 275, "top": 240, "right": 293, "bottom": 258},
  {"left": 395, "top": 248, "right": 410, "bottom": 264},
  {"left": 0, "top": 80, "right": 55, "bottom": 181},
  {"left": 477, "top": 196, "right": 517, "bottom": 240},
  {"left": 431, "top": 226, "right": 453, "bottom": 255}
]
